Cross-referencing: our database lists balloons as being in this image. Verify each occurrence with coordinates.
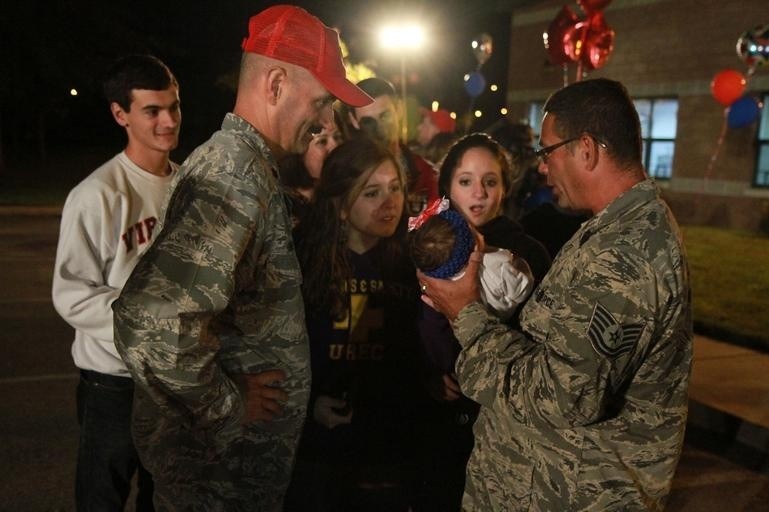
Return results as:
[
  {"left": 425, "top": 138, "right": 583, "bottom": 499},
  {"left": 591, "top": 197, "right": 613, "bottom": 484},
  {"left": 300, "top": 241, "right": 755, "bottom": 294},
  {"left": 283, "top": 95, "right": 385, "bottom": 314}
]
[
  {"left": 723, "top": 96, "right": 759, "bottom": 130},
  {"left": 735, "top": 22, "right": 769, "bottom": 71},
  {"left": 468, "top": 33, "right": 495, "bottom": 66},
  {"left": 576, "top": 1, "right": 609, "bottom": 25},
  {"left": 577, "top": 12, "right": 619, "bottom": 70},
  {"left": 708, "top": 67, "right": 748, "bottom": 107},
  {"left": 540, "top": 4, "right": 592, "bottom": 69}
]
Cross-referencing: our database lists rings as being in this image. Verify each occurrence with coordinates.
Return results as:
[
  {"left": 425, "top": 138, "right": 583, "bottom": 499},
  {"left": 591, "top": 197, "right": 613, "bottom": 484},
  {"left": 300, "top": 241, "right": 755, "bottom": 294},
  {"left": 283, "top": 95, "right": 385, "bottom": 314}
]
[{"left": 421, "top": 284, "right": 429, "bottom": 295}]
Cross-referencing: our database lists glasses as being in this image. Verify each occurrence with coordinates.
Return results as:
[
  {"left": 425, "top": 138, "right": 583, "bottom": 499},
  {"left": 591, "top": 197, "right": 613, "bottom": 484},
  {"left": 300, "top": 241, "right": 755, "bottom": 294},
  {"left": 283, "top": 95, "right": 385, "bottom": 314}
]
[{"left": 535, "top": 133, "right": 608, "bottom": 164}]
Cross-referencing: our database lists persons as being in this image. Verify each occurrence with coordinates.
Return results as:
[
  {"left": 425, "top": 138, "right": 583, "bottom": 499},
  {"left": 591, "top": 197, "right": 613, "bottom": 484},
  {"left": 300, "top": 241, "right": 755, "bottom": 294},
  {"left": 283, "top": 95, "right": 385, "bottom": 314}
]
[
  {"left": 112, "top": 3, "right": 378, "bottom": 511},
  {"left": 281, "top": 129, "right": 420, "bottom": 510},
  {"left": 400, "top": 130, "right": 555, "bottom": 511},
  {"left": 49, "top": 51, "right": 186, "bottom": 511},
  {"left": 283, "top": 79, "right": 594, "bottom": 266},
  {"left": 412, "top": 73, "right": 697, "bottom": 510},
  {"left": 406, "top": 207, "right": 537, "bottom": 325}
]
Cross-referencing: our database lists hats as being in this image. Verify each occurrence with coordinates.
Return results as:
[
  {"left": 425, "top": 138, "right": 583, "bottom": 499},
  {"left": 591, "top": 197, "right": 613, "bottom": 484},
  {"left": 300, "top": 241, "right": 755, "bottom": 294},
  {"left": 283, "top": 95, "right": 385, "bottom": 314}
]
[
  {"left": 416, "top": 105, "right": 458, "bottom": 132},
  {"left": 242, "top": 5, "right": 376, "bottom": 108}
]
